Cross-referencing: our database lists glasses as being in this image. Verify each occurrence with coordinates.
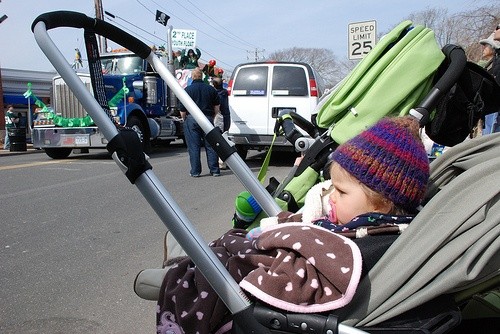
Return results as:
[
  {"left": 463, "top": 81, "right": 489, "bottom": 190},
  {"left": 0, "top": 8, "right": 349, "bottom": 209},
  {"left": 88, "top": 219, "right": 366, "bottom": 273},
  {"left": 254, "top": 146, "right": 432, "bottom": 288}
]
[{"left": 496, "top": 26, "right": 500, "bottom": 30}]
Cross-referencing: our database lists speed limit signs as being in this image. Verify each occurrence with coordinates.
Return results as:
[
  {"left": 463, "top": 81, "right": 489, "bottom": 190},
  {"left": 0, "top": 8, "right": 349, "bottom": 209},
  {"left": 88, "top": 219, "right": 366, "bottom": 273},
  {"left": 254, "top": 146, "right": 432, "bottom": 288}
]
[{"left": 348, "top": 19, "right": 377, "bottom": 60}]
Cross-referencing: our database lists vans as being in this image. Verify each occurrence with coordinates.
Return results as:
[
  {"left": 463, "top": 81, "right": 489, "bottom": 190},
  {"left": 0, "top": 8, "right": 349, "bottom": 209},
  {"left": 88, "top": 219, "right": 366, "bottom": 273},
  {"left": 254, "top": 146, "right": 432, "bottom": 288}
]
[{"left": 226, "top": 61, "right": 326, "bottom": 170}]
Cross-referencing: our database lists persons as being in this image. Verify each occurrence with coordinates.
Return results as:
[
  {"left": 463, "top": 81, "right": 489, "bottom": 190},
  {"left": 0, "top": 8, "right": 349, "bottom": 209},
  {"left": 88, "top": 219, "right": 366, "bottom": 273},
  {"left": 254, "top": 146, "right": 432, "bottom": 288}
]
[
  {"left": 133, "top": 115, "right": 429, "bottom": 303},
  {"left": 476, "top": 23, "right": 500, "bottom": 86},
  {"left": 180, "top": 69, "right": 221, "bottom": 177},
  {"left": 4, "top": 105, "right": 19, "bottom": 149},
  {"left": 209, "top": 78, "right": 231, "bottom": 171},
  {"left": 156, "top": 45, "right": 226, "bottom": 83}
]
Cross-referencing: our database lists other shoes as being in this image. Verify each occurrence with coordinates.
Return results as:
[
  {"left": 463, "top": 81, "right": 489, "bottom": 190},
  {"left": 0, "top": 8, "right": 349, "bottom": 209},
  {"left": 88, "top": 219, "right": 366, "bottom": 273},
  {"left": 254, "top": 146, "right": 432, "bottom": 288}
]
[
  {"left": 163, "top": 229, "right": 186, "bottom": 266},
  {"left": 133, "top": 266, "right": 168, "bottom": 301}
]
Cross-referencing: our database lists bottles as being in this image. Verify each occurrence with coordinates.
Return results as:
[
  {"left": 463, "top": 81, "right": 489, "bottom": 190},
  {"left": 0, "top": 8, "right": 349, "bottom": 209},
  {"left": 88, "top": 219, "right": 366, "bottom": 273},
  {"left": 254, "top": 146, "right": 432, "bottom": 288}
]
[{"left": 232, "top": 190, "right": 263, "bottom": 230}]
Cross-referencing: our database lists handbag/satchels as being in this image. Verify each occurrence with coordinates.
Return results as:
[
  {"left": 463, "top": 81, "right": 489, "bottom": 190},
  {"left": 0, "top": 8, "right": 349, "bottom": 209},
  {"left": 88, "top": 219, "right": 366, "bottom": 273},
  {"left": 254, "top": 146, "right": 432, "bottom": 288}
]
[
  {"left": 425, "top": 40, "right": 499, "bottom": 146},
  {"left": 213, "top": 112, "right": 224, "bottom": 133}
]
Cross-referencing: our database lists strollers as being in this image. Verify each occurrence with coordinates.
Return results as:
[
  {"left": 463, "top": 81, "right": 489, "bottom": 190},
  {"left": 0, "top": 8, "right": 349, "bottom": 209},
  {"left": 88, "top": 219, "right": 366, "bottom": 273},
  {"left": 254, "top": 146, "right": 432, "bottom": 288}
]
[{"left": 31, "top": 10, "right": 500, "bottom": 333}]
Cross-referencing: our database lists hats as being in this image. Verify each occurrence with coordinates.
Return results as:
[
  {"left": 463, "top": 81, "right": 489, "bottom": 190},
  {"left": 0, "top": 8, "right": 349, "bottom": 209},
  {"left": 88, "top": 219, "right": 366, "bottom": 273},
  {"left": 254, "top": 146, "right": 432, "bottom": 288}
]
[
  {"left": 331, "top": 116, "right": 430, "bottom": 214},
  {"left": 479, "top": 33, "right": 500, "bottom": 49}
]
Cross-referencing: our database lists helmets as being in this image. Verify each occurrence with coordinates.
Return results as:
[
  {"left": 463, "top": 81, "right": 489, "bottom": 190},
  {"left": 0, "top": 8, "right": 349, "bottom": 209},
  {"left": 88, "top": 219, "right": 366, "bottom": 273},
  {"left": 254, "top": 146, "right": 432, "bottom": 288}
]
[{"left": 210, "top": 60, "right": 216, "bottom": 65}]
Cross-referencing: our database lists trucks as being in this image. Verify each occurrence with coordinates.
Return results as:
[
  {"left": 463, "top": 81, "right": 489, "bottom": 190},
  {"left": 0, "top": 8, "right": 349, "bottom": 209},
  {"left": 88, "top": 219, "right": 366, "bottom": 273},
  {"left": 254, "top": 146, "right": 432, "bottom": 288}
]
[{"left": 30, "top": 24, "right": 231, "bottom": 159}]
